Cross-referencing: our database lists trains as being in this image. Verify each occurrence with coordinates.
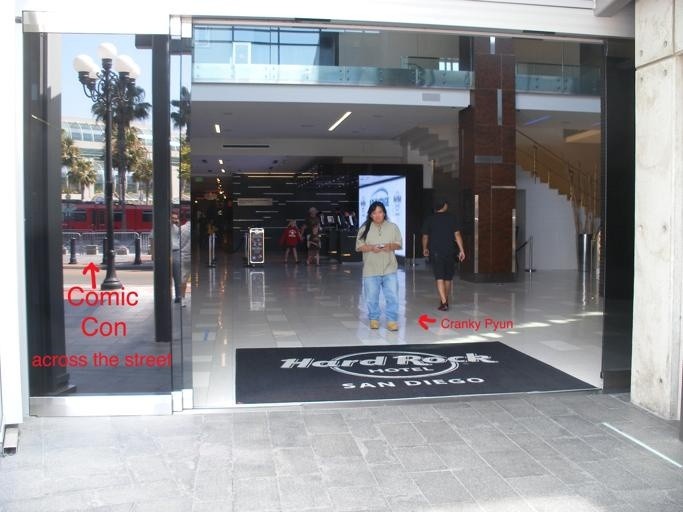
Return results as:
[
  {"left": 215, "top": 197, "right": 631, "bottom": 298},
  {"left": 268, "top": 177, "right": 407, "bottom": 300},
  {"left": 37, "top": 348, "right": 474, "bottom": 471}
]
[{"left": 61, "top": 193, "right": 191, "bottom": 234}]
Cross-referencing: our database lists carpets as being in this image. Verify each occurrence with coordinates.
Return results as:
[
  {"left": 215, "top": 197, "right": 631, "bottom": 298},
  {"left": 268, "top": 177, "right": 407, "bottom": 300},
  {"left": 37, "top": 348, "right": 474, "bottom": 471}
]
[{"left": 236, "top": 340, "right": 603, "bottom": 406}]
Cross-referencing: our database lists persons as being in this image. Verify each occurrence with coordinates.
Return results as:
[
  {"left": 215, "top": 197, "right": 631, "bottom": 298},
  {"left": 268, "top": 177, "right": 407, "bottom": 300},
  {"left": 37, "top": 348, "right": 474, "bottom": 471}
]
[
  {"left": 334, "top": 208, "right": 356, "bottom": 235},
  {"left": 147, "top": 211, "right": 180, "bottom": 304},
  {"left": 278, "top": 221, "right": 304, "bottom": 265},
  {"left": 298, "top": 206, "right": 322, "bottom": 266},
  {"left": 419, "top": 198, "right": 467, "bottom": 312},
  {"left": 178, "top": 212, "right": 191, "bottom": 307},
  {"left": 353, "top": 201, "right": 403, "bottom": 331}
]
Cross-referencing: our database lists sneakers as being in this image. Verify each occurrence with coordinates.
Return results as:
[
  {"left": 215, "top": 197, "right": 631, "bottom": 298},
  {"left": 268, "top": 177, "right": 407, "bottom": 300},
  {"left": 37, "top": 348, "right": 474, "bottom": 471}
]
[
  {"left": 386, "top": 320, "right": 399, "bottom": 331},
  {"left": 370, "top": 320, "right": 380, "bottom": 329},
  {"left": 437, "top": 300, "right": 448, "bottom": 311}
]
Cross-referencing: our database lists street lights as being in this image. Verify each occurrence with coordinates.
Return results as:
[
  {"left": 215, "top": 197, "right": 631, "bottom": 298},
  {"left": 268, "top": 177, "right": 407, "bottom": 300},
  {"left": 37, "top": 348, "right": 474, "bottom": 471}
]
[{"left": 71, "top": 44, "right": 136, "bottom": 289}]
